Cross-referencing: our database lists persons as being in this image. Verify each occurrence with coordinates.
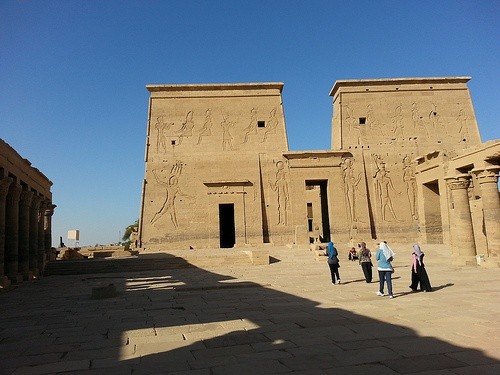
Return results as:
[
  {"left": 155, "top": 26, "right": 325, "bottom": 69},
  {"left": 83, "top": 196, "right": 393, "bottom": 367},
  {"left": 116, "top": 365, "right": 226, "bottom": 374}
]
[
  {"left": 326, "top": 242, "right": 341, "bottom": 285},
  {"left": 349, "top": 247, "right": 358, "bottom": 261},
  {"left": 409, "top": 244, "right": 427, "bottom": 293},
  {"left": 376, "top": 242, "right": 393, "bottom": 299},
  {"left": 358, "top": 242, "right": 373, "bottom": 283}
]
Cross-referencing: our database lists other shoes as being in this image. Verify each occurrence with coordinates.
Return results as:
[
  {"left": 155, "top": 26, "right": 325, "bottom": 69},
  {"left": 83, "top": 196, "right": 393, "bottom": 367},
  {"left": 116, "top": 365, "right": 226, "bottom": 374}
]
[
  {"left": 411, "top": 290, "right": 416, "bottom": 293},
  {"left": 332, "top": 283, "right": 335, "bottom": 285},
  {"left": 337, "top": 279, "right": 340, "bottom": 284},
  {"left": 389, "top": 295, "right": 393, "bottom": 298},
  {"left": 376, "top": 290, "right": 385, "bottom": 296}
]
